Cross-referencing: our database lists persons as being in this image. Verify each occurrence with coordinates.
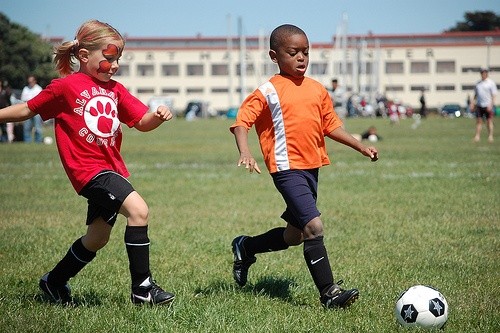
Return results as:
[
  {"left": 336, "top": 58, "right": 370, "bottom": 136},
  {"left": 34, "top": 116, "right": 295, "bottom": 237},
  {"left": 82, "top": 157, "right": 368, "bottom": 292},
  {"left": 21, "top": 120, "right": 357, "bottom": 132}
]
[
  {"left": 0, "top": 20, "right": 175, "bottom": 306},
  {"left": 230, "top": 25, "right": 379, "bottom": 308},
  {"left": 327, "top": 79, "right": 344, "bottom": 118},
  {"left": 0, "top": 75, "right": 43, "bottom": 143},
  {"left": 471, "top": 69, "right": 497, "bottom": 142},
  {"left": 361, "top": 127, "right": 380, "bottom": 140}
]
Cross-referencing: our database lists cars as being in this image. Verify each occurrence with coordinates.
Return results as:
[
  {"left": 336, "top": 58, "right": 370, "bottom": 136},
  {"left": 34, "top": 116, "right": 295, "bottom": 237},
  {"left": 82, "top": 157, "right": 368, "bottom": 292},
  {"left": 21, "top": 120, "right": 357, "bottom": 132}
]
[{"left": 440, "top": 105, "right": 464, "bottom": 118}]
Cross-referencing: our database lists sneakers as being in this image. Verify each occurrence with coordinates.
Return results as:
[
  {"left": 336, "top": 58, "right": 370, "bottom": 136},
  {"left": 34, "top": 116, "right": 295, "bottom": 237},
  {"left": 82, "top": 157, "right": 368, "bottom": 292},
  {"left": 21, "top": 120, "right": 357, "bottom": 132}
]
[
  {"left": 38, "top": 272, "right": 72, "bottom": 304},
  {"left": 130, "top": 277, "right": 175, "bottom": 306},
  {"left": 319, "top": 282, "right": 359, "bottom": 311},
  {"left": 231, "top": 234, "right": 258, "bottom": 286}
]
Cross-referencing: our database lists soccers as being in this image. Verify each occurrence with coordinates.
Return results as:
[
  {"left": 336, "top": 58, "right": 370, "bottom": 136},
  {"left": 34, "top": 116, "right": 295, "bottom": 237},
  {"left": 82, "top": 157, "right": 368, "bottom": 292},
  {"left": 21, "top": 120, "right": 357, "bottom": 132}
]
[{"left": 395, "top": 284, "right": 450, "bottom": 331}]
